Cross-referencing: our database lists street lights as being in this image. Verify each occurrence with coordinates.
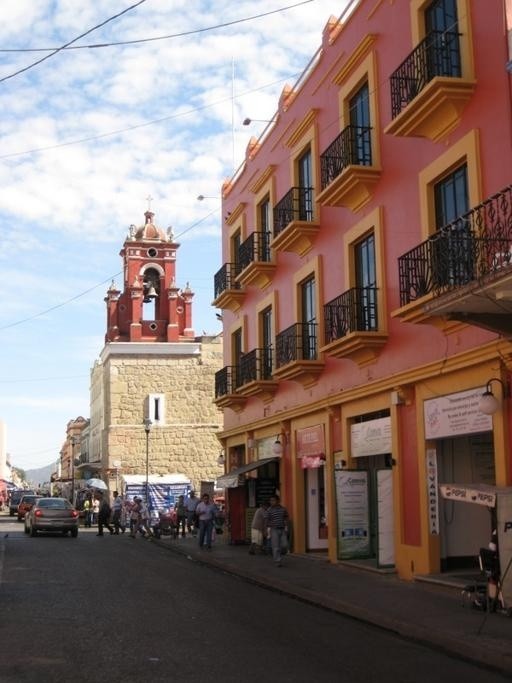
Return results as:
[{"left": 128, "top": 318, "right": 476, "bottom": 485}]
[
  {"left": 143, "top": 418, "right": 153, "bottom": 502},
  {"left": 70, "top": 436, "right": 77, "bottom": 503}
]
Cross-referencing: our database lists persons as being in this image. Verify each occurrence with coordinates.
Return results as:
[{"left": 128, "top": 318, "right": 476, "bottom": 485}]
[
  {"left": 249, "top": 501, "right": 271, "bottom": 556},
  {"left": 184, "top": 490, "right": 200, "bottom": 536},
  {"left": 73, "top": 486, "right": 156, "bottom": 539},
  {"left": 173, "top": 495, "right": 187, "bottom": 537},
  {"left": 266, "top": 494, "right": 289, "bottom": 567},
  {"left": 196, "top": 492, "right": 221, "bottom": 549}
]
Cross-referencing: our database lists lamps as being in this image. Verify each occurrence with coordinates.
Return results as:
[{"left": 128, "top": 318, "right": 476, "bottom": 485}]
[
  {"left": 248, "top": 438, "right": 256, "bottom": 450},
  {"left": 198, "top": 196, "right": 224, "bottom": 200},
  {"left": 243, "top": 119, "right": 279, "bottom": 126},
  {"left": 273, "top": 433, "right": 287, "bottom": 454},
  {"left": 217, "top": 448, "right": 225, "bottom": 465},
  {"left": 479, "top": 378, "right": 506, "bottom": 414}
]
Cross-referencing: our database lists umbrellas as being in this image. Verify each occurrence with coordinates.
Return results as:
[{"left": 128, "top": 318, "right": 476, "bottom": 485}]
[{"left": 87, "top": 477, "right": 109, "bottom": 495}]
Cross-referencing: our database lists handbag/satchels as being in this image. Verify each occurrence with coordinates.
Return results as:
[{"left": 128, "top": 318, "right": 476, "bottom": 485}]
[
  {"left": 142, "top": 509, "right": 149, "bottom": 520},
  {"left": 280, "top": 534, "right": 288, "bottom": 549}
]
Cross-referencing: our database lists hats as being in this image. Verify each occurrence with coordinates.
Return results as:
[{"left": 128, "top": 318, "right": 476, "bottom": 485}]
[{"left": 136, "top": 497, "right": 141, "bottom": 499}]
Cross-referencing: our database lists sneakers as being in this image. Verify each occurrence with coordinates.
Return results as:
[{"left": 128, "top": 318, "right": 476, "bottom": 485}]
[
  {"left": 274, "top": 562, "right": 281, "bottom": 567},
  {"left": 96, "top": 528, "right": 125, "bottom": 536}
]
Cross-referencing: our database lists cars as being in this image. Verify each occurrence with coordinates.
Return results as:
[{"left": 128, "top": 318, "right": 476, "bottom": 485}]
[{"left": 9, "top": 490, "right": 78, "bottom": 537}]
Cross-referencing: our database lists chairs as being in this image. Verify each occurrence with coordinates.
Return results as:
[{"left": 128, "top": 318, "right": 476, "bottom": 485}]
[{"left": 454, "top": 549, "right": 494, "bottom": 609}]
